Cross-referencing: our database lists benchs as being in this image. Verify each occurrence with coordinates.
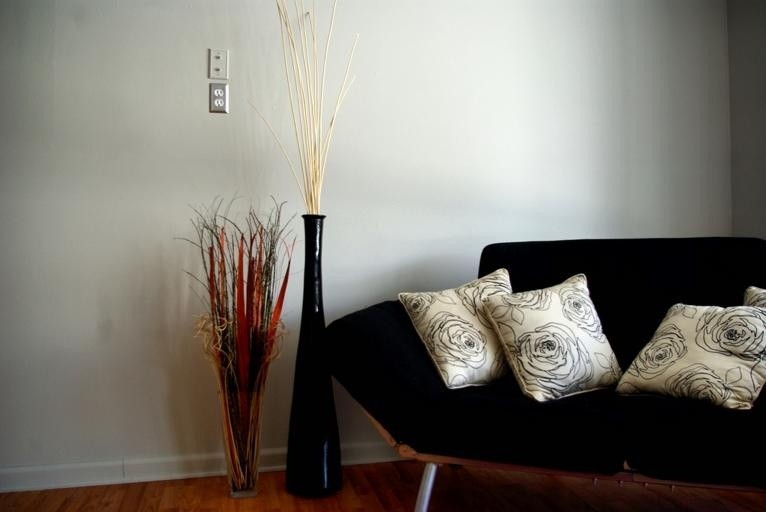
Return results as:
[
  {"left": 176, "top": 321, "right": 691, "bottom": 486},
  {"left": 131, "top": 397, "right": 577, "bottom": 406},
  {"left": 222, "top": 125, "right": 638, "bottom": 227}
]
[{"left": 322, "top": 238, "right": 766, "bottom": 512}]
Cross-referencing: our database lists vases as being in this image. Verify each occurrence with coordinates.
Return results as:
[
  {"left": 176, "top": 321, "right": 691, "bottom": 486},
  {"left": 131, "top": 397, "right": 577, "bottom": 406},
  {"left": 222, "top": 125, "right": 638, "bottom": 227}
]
[
  {"left": 203, "top": 328, "right": 283, "bottom": 501},
  {"left": 282, "top": 213, "right": 344, "bottom": 497}
]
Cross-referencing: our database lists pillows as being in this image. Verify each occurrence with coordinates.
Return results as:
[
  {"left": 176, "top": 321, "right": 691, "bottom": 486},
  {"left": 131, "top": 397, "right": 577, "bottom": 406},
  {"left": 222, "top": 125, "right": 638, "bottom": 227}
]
[
  {"left": 482, "top": 273, "right": 622, "bottom": 403},
  {"left": 616, "top": 304, "right": 766, "bottom": 410},
  {"left": 399, "top": 268, "right": 514, "bottom": 391},
  {"left": 743, "top": 285, "right": 766, "bottom": 307}
]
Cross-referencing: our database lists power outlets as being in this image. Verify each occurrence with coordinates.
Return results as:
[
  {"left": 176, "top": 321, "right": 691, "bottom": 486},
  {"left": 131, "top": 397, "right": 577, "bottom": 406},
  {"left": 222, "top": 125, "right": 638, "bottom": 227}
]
[{"left": 209, "top": 83, "right": 228, "bottom": 114}]
